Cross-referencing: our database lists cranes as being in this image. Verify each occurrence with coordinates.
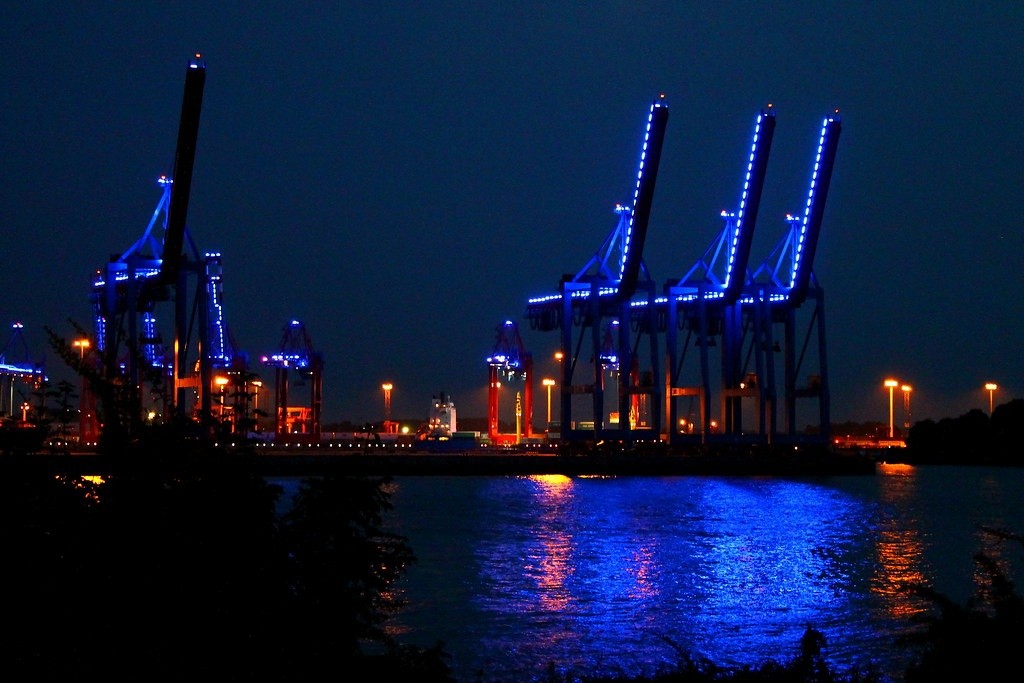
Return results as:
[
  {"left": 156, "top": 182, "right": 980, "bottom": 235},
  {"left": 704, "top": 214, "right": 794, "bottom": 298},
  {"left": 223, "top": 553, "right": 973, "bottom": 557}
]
[
  {"left": 529, "top": 86, "right": 841, "bottom": 456},
  {"left": 95, "top": 49, "right": 241, "bottom": 453}
]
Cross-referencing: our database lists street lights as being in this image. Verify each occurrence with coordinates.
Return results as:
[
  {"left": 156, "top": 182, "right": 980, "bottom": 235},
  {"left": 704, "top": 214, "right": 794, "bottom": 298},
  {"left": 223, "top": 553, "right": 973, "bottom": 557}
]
[
  {"left": 214, "top": 375, "right": 229, "bottom": 421},
  {"left": 382, "top": 383, "right": 394, "bottom": 420},
  {"left": 251, "top": 381, "right": 262, "bottom": 431},
  {"left": 899, "top": 385, "right": 913, "bottom": 438},
  {"left": 542, "top": 377, "right": 556, "bottom": 422},
  {"left": 883, "top": 379, "right": 900, "bottom": 438},
  {"left": 984, "top": 382, "right": 997, "bottom": 416}
]
